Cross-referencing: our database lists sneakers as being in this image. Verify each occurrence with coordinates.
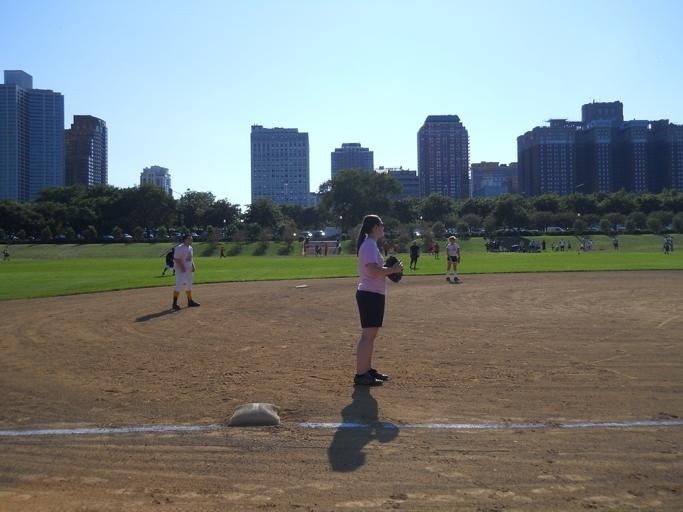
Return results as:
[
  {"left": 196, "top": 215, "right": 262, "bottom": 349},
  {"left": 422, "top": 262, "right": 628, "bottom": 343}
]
[{"left": 445, "top": 276, "right": 461, "bottom": 280}]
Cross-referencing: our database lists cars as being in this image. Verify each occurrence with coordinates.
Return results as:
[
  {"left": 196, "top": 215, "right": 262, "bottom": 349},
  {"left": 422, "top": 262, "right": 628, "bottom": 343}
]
[
  {"left": 310, "top": 227, "right": 337, "bottom": 241},
  {"left": 293, "top": 230, "right": 310, "bottom": 243},
  {"left": 519, "top": 225, "right": 539, "bottom": 235},
  {"left": 545, "top": 226, "right": 565, "bottom": 233}
]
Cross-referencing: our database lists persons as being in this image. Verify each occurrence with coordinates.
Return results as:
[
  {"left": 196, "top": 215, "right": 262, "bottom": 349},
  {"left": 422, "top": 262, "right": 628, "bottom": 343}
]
[
  {"left": 1, "top": 245, "right": 10, "bottom": 263},
  {"left": 353, "top": 214, "right": 404, "bottom": 385},
  {"left": 445, "top": 236, "right": 463, "bottom": 283},
  {"left": 218, "top": 245, "right": 226, "bottom": 258},
  {"left": 433, "top": 241, "right": 439, "bottom": 258},
  {"left": 484, "top": 236, "right": 593, "bottom": 255},
  {"left": 159, "top": 248, "right": 175, "bottom": 276},
  {"left": 612, "top": 237, "right": 618, "bottom": 251},
  {"left": 172, "top": 235, "right": 199, "bottom": 310},
  {"left": 392, "top": 240, "right": 398, "bottom": 255},
  {"left": 662, "top": 234, "right": 673, "bottom": 255},
  {"left": 299, "top": 235, "right": 340, "bottom": 257},
  {"left": 383, "top": 240, "right": 390, "bottom": 256},
  {"left": 408, "top": 240, "right": 420, "bottom": 269}
]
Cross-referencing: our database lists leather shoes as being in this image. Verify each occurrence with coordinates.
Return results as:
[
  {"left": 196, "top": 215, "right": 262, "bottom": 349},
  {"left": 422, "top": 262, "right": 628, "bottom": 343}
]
[
  {"left": 188, "top": 301, "right": 200, "bottom": 306},
  {"left": 354, "top": 368, "right": 391, "bottom": 386},
  {"left": 172, "top": 304, "right": 181, "bottom": 310}
]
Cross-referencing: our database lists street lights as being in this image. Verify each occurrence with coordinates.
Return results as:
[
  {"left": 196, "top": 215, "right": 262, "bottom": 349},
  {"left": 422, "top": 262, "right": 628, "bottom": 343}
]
[{"left": 338, "top": 216, "right": 343, "bottom": 255}]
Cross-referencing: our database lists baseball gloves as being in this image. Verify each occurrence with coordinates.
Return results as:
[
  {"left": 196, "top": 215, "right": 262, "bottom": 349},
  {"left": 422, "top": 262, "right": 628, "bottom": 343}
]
[{"left": 384, "top": 256, "right": 403, "bottom": 282}]
